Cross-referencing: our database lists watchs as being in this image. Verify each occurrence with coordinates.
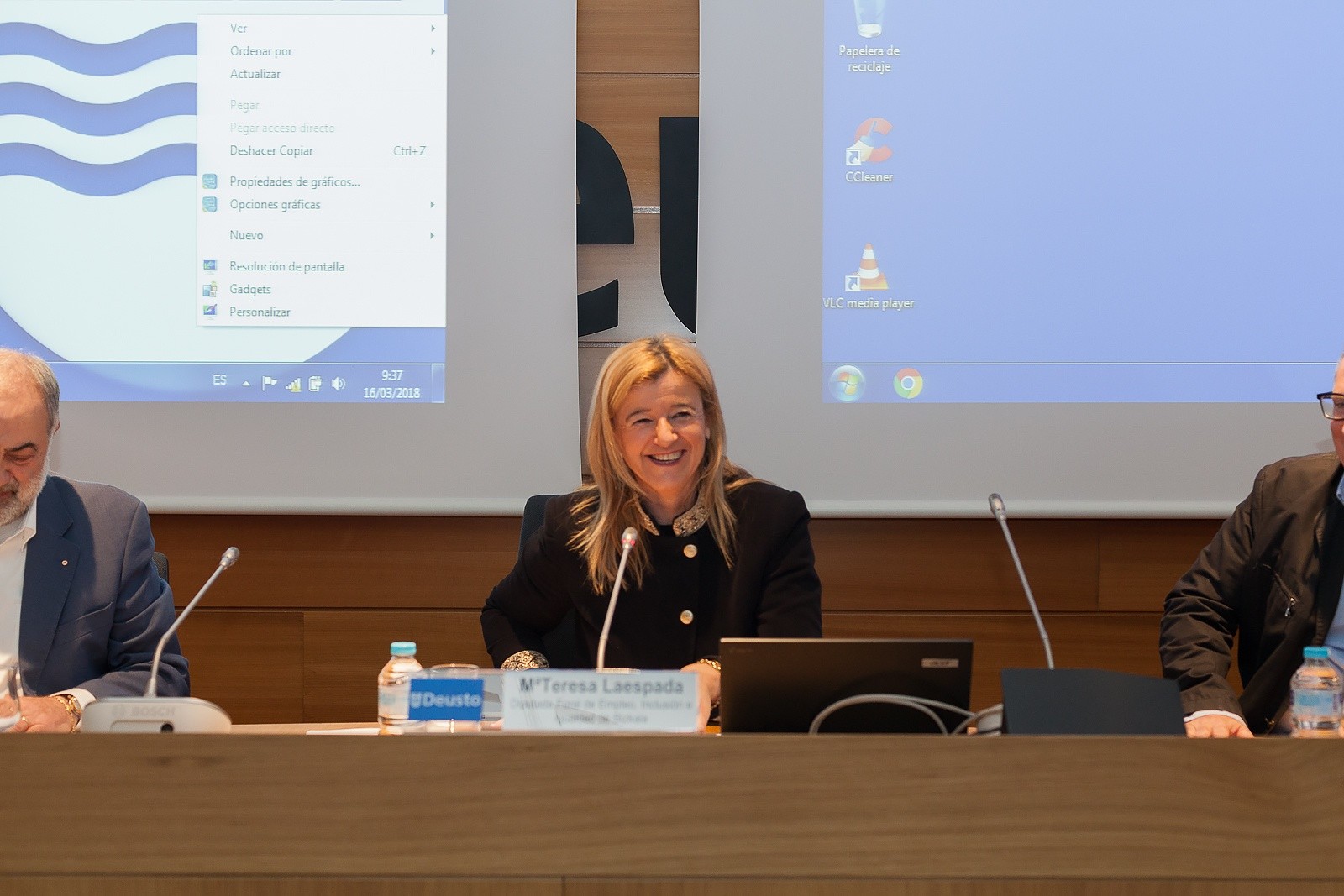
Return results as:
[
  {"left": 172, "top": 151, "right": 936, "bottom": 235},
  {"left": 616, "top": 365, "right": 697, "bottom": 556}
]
[{"left": 62, "top": 695, "right": 81, "bottom": 721}]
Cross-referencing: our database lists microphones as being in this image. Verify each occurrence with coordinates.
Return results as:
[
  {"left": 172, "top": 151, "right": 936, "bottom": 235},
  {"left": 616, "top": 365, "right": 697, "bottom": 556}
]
[
  {"left": 989, "top": 492, "right": 1055, "bottom": 668},
  {"left": 598, "top": 527, "right": 637, "bottom": 669},
  {"left": 82, "top": 547, "right": 242, "bottom": 736}
]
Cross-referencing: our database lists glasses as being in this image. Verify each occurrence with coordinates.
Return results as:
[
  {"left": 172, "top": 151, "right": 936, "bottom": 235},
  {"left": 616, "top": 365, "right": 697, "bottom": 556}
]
[{"left": 1316, "top": 392, "right": 1344, "bottom": 421}]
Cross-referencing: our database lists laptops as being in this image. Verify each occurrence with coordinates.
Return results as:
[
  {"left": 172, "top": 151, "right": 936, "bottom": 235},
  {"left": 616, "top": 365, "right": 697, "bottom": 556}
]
[{"left": 719, "top": 638, "right": 975, "bottom": 733}]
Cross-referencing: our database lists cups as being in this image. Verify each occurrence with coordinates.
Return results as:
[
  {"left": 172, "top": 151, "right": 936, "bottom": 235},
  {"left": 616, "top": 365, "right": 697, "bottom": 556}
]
[
  {"left": 0, "top": 667, "right": 22, "bottom": 731},
  {"left": 427, "top": 663, "right": 483, "bottom": 733}
]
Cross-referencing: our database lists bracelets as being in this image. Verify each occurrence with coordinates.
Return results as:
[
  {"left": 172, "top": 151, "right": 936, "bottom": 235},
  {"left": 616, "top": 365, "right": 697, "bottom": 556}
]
[
  {"left": 696, "top": 658, "right": 721, "bottom": 710},
  {"left": 52, "top": 696, "right": 76, "bottom": 734}
]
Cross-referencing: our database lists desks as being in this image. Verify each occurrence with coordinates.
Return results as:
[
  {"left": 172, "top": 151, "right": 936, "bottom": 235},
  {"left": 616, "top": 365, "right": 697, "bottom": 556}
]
[{"left": 0, "top": 724, "right": 1344, "bottom": 894}]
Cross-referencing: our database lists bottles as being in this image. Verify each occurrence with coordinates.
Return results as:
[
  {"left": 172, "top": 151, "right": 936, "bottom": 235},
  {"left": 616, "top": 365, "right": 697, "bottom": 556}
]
[
  {"left": 377, "top": 640, "right": 424, "bottom": 734},
  {"left": 1290, "top": 646, "right": 1344, "bottom": 739}
]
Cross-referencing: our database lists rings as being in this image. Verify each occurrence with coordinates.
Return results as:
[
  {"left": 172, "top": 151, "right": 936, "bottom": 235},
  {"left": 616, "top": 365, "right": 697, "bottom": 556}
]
[
  {"left": 9, "top": 707, "right": 15, "bottom": 714},
  {"left": 20, "top": 716, "right": 30, "bottom": 728}
]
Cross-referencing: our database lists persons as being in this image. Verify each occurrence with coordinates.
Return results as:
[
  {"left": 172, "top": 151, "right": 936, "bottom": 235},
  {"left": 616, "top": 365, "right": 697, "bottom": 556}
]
[
  {"left": 1158, "top": 351, "right": 1344, "bottom": 739},
  {"left": 480, "top": 332, "right": 823, "bottom": 731},
  {"left": 0, "top": 346, "right": 190, "bottom": 734}
]
